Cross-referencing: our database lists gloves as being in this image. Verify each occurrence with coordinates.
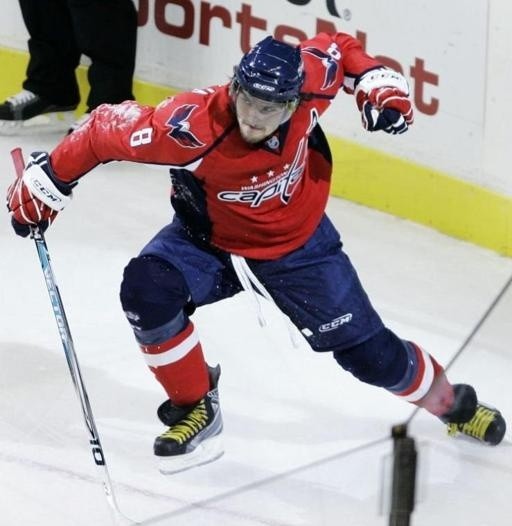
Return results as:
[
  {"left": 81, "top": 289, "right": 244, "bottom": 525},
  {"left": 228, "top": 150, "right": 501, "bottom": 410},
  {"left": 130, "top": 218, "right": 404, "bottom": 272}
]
[
  {"left": 353, "top": 62, "right": 417, "bottom": 135},
  {"left": 6, "top": 148, "right": 78, "bottom": 238}
]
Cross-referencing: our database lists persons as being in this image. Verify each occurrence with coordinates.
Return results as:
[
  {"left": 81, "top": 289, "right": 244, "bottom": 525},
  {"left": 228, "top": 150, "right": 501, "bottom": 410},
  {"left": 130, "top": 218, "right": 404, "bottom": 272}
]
[
  {"left": 6, "top": 32, "right": 507, "bottom": 455},
  {"left": 0, "top": 0, "right": 135, "bottom": 134}
]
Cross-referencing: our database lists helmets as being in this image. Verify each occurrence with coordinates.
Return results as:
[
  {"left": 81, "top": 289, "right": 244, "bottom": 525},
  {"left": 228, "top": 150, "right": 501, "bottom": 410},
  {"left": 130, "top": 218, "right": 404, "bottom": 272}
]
[{"left": 232, "top": 33, "right": 307, "bottom": 104}]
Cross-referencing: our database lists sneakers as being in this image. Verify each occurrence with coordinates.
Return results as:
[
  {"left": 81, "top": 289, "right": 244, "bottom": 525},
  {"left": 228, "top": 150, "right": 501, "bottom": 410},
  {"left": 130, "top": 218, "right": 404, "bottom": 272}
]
[
  {"left": 151, "top": 389, "right": 227, "bottom": 457},
  {"left": 435, "top": 385, "right": 506, "bottom": 449},
  {"left": 0, "top": 84, "right": 81, "bottom": 120}
]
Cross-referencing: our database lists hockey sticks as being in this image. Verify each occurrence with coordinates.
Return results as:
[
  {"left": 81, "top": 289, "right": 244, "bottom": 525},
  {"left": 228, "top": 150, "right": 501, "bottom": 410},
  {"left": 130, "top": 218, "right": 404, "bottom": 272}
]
[{"left": 10, "top": 148, "right": 141, "bottom": 526}]
